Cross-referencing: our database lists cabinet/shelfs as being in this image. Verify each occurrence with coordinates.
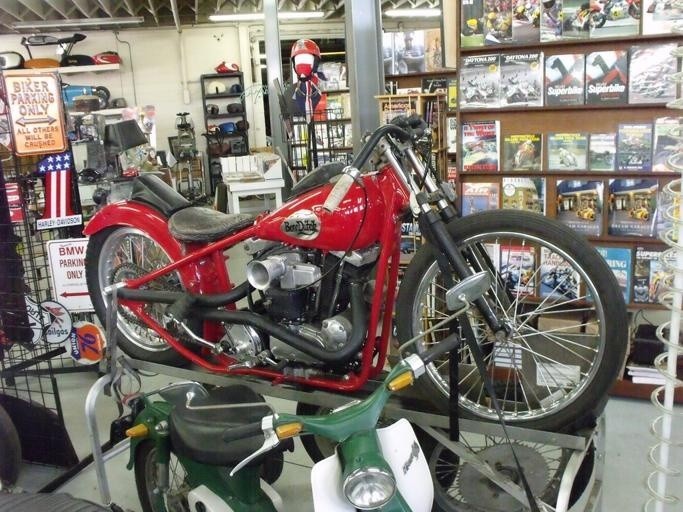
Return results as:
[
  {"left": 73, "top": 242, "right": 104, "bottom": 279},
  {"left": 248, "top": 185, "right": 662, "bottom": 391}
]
[
  {"left": 199, "top": 71, "right": 249, "bottom": 198},
  {"left": 373, "top": 92, "right": 450, "bottom": 266},
  {"left": 286, "top": 86, "right": 352, "bottom": 188},
  {"left": 454, "top": 0, "right": 683, "bottom": 409},
  {"left": 3, "top": 60, "right": 133, "bottom": 119}
]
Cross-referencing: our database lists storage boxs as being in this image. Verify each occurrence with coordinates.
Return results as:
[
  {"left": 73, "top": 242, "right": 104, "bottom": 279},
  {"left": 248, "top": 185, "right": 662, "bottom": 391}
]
[
  {"left": 539, "top": 303, "right": 584, "bottom": 336},
  {"left": 580, "top": 310, "right": 634, "bottom": 358}
]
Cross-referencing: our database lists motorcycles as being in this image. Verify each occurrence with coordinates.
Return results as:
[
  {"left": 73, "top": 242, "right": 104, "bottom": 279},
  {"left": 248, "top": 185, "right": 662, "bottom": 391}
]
[
  {"left": 649, "top": 269, "right": 675, "bottom": 303},
  {"left": 83, "top": 101, "right": 627, "bottom": 428},
  {"left": 496, "top": 259, "right": 580, "bottom": 300},
  {"left": 464, "top": 0, "right": 643, "bottom": 49},
  {"left": 589, "top": 150, "right": 614, "bottom": 167},
  {"left": 554, "top": 145, "right": 579, "bottom": 168},
  {"left": 508, "top": 144, "right": 539, "bottom": 169},
  {"left": 87, "top": 332, "right": 459, "bottom": 510},
  {"left": 460, "top": 67, "right": 496, "bottom": 105},
  {"left": 502, "top": 68, "right": 540, "bottom": 102}
]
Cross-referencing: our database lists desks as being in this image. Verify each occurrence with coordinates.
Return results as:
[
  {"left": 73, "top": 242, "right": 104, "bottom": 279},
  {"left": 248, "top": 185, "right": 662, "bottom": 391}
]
[{"left": 217, "top": 171, "right": 285, "bottom": 220}]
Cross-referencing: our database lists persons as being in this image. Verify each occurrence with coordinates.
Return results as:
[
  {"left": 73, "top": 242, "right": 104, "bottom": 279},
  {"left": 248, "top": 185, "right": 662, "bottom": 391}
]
[
  {"left": 427, "top": 35, "right": 442, "bottom": 70},
  {"left": 394, "top": 30, "right": 425, "bottom": 75}
]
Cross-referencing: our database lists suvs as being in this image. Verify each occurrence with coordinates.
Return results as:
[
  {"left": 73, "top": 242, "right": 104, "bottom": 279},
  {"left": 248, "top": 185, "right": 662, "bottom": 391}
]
[{"left": 385, "top": 46, "right": 427, "bottom": 75}]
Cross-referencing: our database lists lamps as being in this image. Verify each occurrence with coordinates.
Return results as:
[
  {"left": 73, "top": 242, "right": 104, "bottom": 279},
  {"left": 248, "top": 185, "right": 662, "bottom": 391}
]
[
  {"left": 99, "top": 118, "right": 149, "bottom": 182},
  {"left": 9, "top": 16, "right": 146, "bottom": 31}
]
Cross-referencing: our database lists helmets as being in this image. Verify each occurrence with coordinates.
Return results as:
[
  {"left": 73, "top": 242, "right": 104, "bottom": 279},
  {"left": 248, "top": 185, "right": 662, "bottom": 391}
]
[
  {"left": 214, "top": 61, "right": 239, "bottom": 75},
  {"left": 289, "top": 37, "right": 322, "bottom": 61},
  {"left": 206, "top": 103, "right": 219, "bottom": 115},
  {"left": 207, "top": 82, "right": 225, "bottom": 95},
  {"left": 230, "top": 81, "right": 242, "bottom": 93},
  {"left": 206, "top": 120, "right": 250, "bottom": 137},
  {"left": 226, "top": 102, "right": 244, "bottom": 113}
]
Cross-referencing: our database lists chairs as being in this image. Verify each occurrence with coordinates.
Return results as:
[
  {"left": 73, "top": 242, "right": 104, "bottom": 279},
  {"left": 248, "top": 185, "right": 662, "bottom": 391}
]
[{"left": 0, "top": 404, "right": 113, "bottom": 512}]
